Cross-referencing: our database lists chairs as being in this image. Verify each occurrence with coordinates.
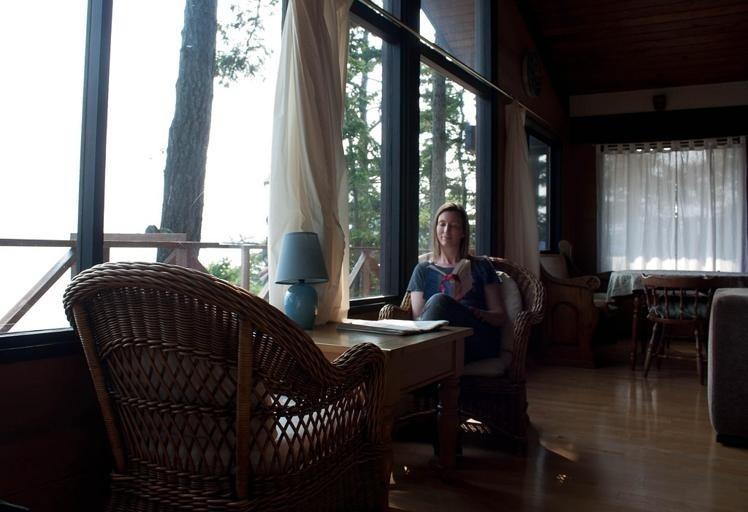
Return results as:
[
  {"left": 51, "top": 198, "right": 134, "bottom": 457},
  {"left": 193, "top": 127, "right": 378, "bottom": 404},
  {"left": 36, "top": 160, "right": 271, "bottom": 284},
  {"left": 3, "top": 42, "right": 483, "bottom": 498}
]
[
  {"left": 537, "top": 241, "right": 615, "bottom": 366},
  {"left": 640, "top": 277, "right": 719, "bottom": 384},
  {"left": 378, "top": 256, "right": 544, "bottom": 432},
  {"left": 62, "top": 261, "right": 394, "bottom": 512}
]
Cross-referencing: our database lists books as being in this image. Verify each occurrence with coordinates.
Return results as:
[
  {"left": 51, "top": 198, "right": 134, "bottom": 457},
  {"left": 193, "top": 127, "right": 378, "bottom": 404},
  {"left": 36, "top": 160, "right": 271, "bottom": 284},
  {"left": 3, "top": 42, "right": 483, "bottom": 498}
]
[{"left": 421, "top": 259, "right": 472, "bottom": 302}]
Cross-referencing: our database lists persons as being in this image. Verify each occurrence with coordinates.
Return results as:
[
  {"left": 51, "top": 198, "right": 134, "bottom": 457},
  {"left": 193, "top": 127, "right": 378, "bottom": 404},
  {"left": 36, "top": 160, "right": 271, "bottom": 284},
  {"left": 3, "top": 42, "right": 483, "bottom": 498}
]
[{"left": 407, "top": 203, "right": 507, "bottom": 366}]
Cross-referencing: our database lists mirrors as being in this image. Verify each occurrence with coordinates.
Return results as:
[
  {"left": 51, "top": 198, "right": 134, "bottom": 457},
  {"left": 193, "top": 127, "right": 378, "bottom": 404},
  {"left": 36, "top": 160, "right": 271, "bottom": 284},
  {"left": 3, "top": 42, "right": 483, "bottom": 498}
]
[{"left": 524, "top": 126, "right": 555, "bottom": 253}]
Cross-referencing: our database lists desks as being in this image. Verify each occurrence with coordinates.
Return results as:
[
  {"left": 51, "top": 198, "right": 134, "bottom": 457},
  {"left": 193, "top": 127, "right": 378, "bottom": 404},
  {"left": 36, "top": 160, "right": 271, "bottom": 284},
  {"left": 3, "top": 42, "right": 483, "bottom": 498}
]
[
  {"left": 306, "top": 320, "right": 473, "bottom": 484},
  {"left": 605, "top": 270, "right": 748, "bottom": 368}
]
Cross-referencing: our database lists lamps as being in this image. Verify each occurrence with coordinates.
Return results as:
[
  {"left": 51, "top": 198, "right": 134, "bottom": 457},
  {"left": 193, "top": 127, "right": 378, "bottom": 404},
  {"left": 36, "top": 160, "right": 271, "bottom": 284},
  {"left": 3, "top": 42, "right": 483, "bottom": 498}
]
[{"left": 276, "top": 231, "right": 331, "bottom": 330}]
